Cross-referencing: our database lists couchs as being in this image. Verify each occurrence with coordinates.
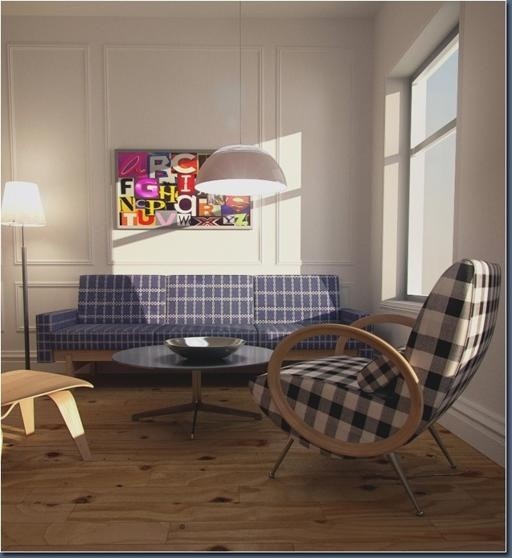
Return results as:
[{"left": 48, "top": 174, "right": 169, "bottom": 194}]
[{"left": 36, "top": 274, "right": 373, "bottom": 391}]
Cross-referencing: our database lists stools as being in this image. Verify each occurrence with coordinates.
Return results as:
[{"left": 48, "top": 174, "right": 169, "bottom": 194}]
[{"left": 1, "top": 370, "right": 95, "bottom": 462}]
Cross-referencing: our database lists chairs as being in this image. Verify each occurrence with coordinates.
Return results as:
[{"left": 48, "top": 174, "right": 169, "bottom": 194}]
[{"left": 247, "top": 257, "right": 502, "bottom": 517}]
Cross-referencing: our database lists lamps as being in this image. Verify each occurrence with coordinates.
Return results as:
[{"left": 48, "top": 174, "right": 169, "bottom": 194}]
[
  {"left": 193, "top": 1, "right": 287, "bottom": 197},
  {"left": 1, "top": 181, "right": 48, "bottom": 370}
]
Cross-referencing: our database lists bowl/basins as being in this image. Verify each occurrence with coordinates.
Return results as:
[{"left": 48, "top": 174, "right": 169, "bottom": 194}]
[{"left": 163, "top": 336, "right": 247, "bottom": 361}]
